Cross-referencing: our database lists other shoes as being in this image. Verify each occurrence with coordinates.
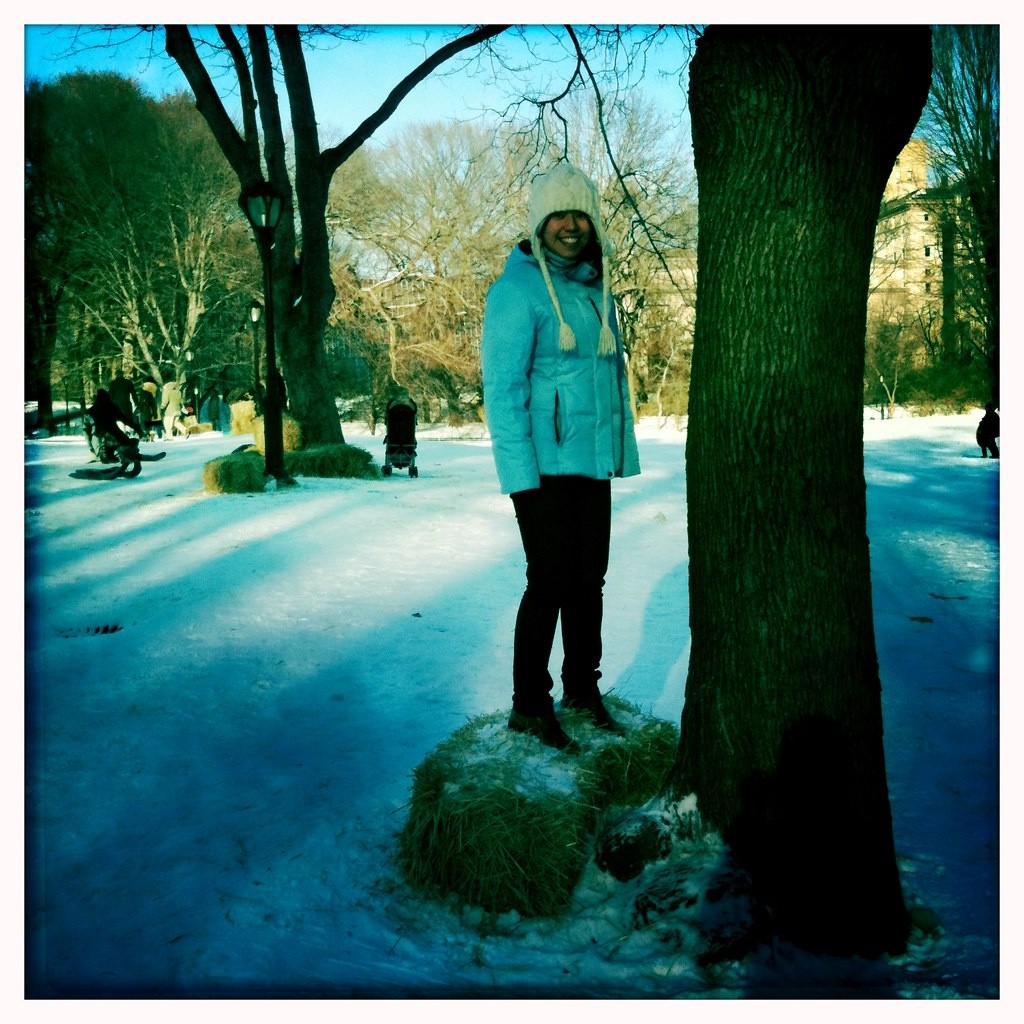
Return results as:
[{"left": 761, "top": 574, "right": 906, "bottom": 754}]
[
  {"left": 561, "top": 687, "right": 633, "bottom": 738},
  {"left": 508, "top": 707, "right": 582, "bottom": 756},
  {"left": 164, "top": 435, "right": 174, "bottom": 441},
  {"left": 185, "top": 429, "right": 192, "bottom": 440}
]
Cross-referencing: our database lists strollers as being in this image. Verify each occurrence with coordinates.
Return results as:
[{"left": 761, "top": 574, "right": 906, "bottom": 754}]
[{"left": 381, "top": 397, "right": 420, "bottom": 479}]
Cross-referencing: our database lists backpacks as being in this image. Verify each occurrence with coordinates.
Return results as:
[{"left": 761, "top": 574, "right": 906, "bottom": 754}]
[{"left": 976, "top": 419, "right": 992, "bottom": 448}]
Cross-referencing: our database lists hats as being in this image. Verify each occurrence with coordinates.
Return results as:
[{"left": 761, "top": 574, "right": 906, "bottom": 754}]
[
  {"left": 985, "top": 401, "right": 996, "bottom": 412},
  {"left": 94, "top": 388, "right": 108, "bottom": 399},
  {"left": 114, "top": 370, "right": 126, "bottom": 378},
  {"left": 528, "top": 163, "right": 617, "bottom": 358}
]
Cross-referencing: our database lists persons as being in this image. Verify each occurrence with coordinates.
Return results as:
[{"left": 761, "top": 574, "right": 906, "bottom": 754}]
[
  {"left": 84, "top": 369, "right": 191, "bottom": 458},
  {"left": 482, "top": 164, "right": 641, "bottom": 754},
  {"left": 981, "top": 402, "right": 999, "bottom": 458}
]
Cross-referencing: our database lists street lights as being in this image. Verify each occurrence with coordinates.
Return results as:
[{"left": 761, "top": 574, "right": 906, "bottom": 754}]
[
  {"left": 247, "top": 298, "right": 263, "bottom": 416},
  {"left": 236, "top": 171, "right": 297, "bottom": 486}
]
